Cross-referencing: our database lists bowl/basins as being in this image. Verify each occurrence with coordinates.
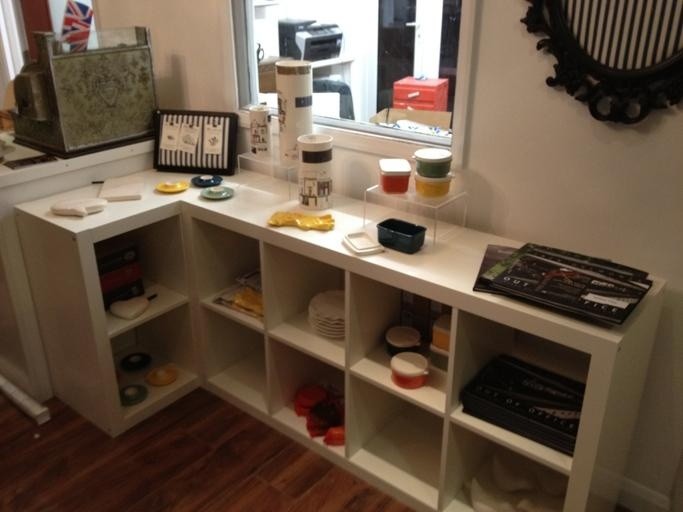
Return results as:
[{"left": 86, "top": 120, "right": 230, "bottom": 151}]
[
  {"left": 411, "top": 148, "right": 453, "bottom": 196},
  {"left": 383, "top": 325, "right": 428, "bottom": 387}
]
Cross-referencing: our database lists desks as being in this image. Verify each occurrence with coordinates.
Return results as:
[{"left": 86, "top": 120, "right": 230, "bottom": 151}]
[{"left": 254, "top": 55, "right": 353, "bottom": 94}]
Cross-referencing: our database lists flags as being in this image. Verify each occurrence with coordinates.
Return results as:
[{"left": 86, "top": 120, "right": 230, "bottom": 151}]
[{"left": 59, "top": 0, "right": 94, "bottom": 56}]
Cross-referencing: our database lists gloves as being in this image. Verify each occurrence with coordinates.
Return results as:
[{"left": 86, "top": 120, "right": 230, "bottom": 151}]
[{"left": 269, "top": 211, "right": 335, "bottom": 231}]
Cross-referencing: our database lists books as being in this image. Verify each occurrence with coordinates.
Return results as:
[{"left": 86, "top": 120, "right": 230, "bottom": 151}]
[
  {"left": 480, "top": 240, "right": 656, "bottom": 325},
  {"left": 459, "top": 348, "right": 586, "bottom": 458},
  {"left": 473, "top": 243, "right": 651, "bottom": 301}
]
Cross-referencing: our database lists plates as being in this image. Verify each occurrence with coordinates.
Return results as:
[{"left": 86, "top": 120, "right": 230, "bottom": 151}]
[
  {"left": 308, "top": 290, "right": 344, "bottom": 338},
  {"left": 155, "top": 175, "right": 235, "bottom": 200},
  {"left": 343, "top": 231, "right": 384, "bottom": 256},
  {"left": 119, "top": 353, "right": 180, "bottom": 405}
]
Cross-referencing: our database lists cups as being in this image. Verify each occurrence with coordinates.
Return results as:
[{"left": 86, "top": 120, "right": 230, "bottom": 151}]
[
  {"left": 274, "top": 61, "right": 313, "bottom": 167},
  {"left": 297, "top": 134, "right": 334, "bottom": 210},
  {"left": 249, "top": 106, "right": 272, "bottom": 160}
]
